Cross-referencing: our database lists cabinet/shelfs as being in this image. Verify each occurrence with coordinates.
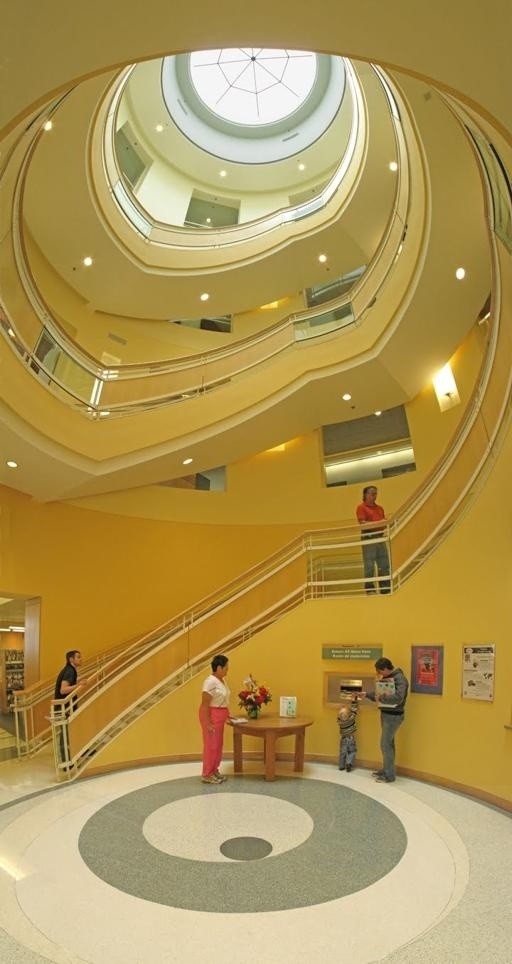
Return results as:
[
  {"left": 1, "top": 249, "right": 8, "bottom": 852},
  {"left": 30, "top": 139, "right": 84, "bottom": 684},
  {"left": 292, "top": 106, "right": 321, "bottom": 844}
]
[{"left": 0, "top": 649, "right": 24, "bottom": 714}]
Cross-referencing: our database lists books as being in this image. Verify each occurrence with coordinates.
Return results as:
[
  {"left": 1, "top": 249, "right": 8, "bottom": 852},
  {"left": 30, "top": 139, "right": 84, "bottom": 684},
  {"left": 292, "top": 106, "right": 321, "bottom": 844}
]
[
  {"left": 229, "top": 718, "right": 248, "bottom": 725},
  {"left": 279, "top": 696, "right": 297, "bottom": 717},
  {"left": 374, "top": 677, "right": 396, "bottom": 709}
]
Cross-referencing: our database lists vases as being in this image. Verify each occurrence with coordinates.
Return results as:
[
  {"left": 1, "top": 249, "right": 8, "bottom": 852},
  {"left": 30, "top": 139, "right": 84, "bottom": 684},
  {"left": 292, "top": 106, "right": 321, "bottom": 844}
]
[{"left": 248, "top": 705, "right": 256, "bottom": 718}]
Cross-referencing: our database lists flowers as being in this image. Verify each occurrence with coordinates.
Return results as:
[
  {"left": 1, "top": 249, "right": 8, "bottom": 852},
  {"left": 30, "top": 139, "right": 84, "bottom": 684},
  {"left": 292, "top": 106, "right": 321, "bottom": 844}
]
[{"left": 238, "top": 674, "right": 273, "bottom": 718}]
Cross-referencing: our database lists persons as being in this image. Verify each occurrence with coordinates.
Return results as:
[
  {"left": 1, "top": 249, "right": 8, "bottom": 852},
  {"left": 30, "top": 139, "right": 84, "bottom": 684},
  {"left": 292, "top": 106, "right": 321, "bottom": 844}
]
[
  {"left": 358, "top": 657, "right": 409, "bottom": 783},
  {"left": 54, "top": 650, "right": 98, "bottom": 772},
  {"left": 197, "top": 653, "right": 239, "bottom": 786},
  {"left": 335, "top": 692, "right": 360, "bottom": 773},
  {"left": 355, "top": 482, "right": 391, "bottom": 594}
]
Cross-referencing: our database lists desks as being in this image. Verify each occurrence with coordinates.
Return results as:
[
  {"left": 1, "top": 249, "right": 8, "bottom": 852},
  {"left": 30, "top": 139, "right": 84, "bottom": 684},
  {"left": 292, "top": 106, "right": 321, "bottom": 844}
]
[{"left": 225, "top": 713, "right": 312, "bottom": 781}]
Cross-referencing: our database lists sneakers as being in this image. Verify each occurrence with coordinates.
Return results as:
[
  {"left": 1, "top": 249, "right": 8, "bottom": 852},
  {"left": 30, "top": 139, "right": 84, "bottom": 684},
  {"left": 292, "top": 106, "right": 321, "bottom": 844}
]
[
  {"left": 201, "top": 769, "right": 227, "bottom": 785},
  {"left": 338, "top": 763, "right": 396, "bottom": 784}
]
